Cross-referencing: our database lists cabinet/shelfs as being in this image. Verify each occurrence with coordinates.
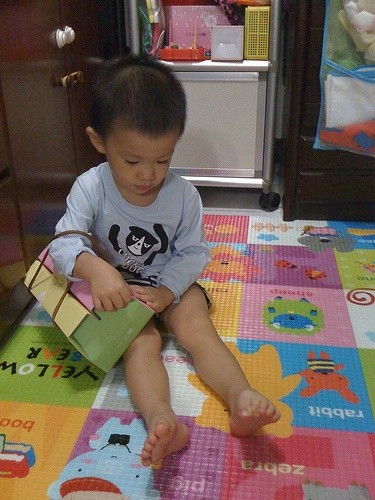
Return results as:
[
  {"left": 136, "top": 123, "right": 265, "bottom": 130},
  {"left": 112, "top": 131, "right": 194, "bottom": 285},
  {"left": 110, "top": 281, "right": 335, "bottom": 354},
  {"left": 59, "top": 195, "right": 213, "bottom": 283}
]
[{"left": 279, "top": 0, "right": 374, "bottom": 223}]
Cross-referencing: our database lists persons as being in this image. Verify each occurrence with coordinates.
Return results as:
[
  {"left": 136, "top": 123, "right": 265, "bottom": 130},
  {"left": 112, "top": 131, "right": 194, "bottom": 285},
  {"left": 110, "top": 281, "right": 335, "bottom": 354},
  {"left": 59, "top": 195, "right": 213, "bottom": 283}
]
[{"left": 47, "top": 51, "right": 282, "bottom": 468}]
